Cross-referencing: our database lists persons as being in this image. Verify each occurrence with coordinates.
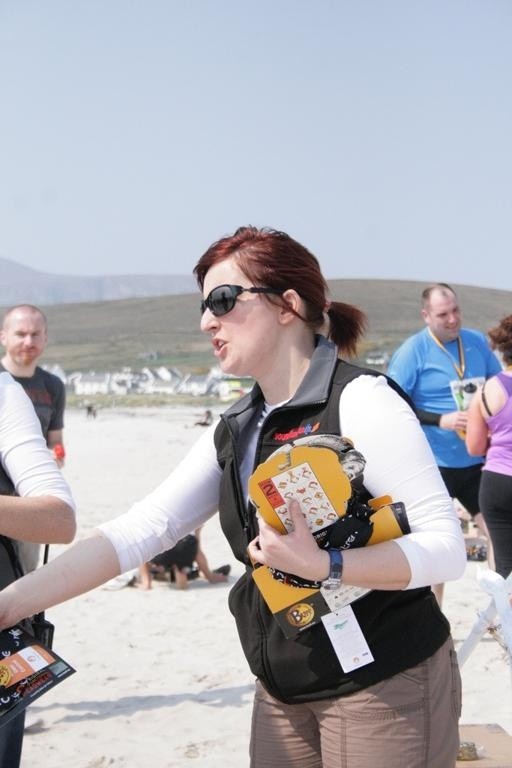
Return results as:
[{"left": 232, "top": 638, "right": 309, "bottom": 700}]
[
  {"left": 464, "top": 317, "right": 512, "bottom": 658},
  {"left": 0, "top": 371, "right": 76, "bottom": 768},
  {"left": 374, "top": 283, "right": 501, "bottom": 559},
  {"left": 135, "top": 527, "right": 224, "bottom": 591},
  {"left": 0, "top": 220, "right": 466, "bottom": 768},
  {"left": 1, "top": 301, "right": 66, "bottom": 576}
]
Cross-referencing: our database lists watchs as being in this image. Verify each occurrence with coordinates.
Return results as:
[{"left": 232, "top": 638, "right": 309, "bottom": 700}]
[{"left": 320, "top": 547, "right": 343, "bottom": 594}]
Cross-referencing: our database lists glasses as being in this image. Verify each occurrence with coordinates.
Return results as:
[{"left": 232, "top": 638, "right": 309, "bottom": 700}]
[{"left": 200, "top": 284, "right": 285, "bottom": 315}]
